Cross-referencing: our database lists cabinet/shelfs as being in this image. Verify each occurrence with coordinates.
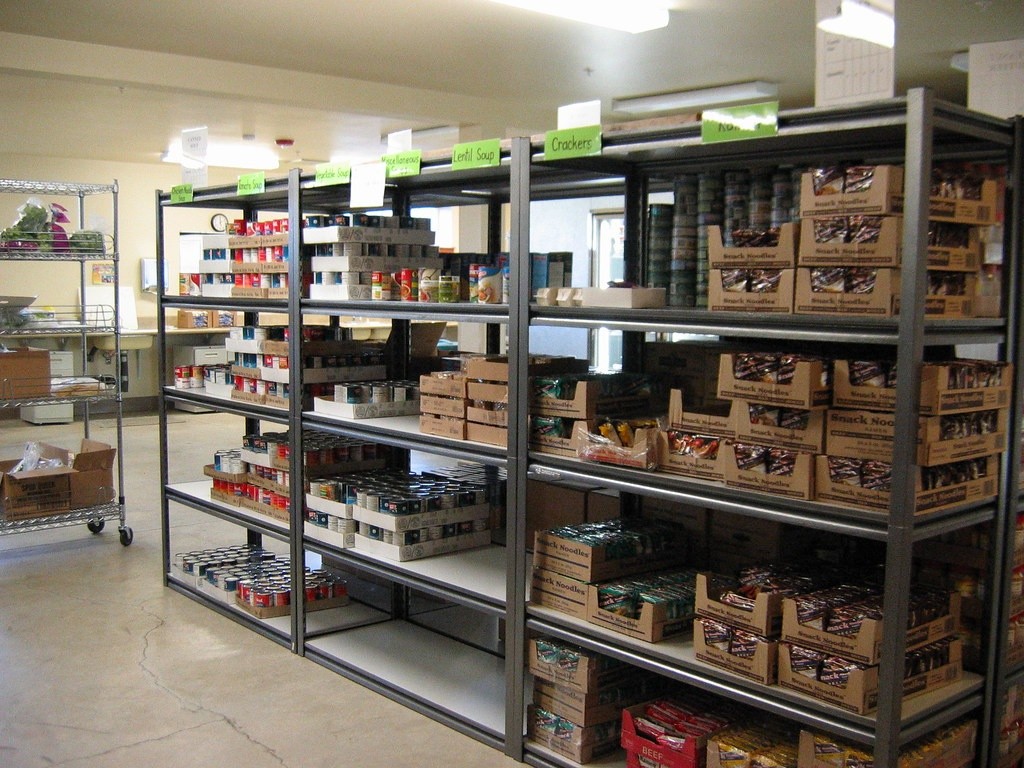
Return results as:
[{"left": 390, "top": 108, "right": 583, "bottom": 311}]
[
  {"left": 514, "top": 88, "right": 1024, "bottom": 768},
  {"left": 294, "top": 133, "right": 522, "bottom": 758},
  {"left": 20, "top": 350, "right": 74, "bottom": 424},
  {"left": 0, "top": 177, "right": 134, "bottom": 547},
  {"left": 149, "top": 167, "right": 296, "bottom": 652},
  {"left": 173, "top": 345, "right": 227, "bottom": 413}
]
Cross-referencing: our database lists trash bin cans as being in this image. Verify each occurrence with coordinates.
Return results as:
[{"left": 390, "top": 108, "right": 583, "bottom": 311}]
[{"left": 437, "top": 339, "right": 458, "bottom": 370}]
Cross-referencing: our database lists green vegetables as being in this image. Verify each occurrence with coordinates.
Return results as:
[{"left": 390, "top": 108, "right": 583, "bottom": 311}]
[
  {"left": 0, "top": 204, "right": 53, "bottom": 252},
  {"left": 69, "top": 232, "right": 104, "bottom": 254}
]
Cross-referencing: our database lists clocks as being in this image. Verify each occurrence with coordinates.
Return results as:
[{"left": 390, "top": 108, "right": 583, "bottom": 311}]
[{"left": 211, "top": 214, "right": 229, "bottom": 232}]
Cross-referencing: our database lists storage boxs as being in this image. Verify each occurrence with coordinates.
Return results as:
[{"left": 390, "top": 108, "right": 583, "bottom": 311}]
[
  {"left": 177, "top": 309, "right": 238, "bottom": 329},
  {"left": 178, "top": 161, "right": 1024, "bottom": 768},
  {"left": 0, "top": 456, "right": 79, "bottom": 520},
  {"left": 38, "top": 438, "right": 117, "bottom": 509},
  {"left": 0, "top": 346, "right": 51, "bottom": 398}
]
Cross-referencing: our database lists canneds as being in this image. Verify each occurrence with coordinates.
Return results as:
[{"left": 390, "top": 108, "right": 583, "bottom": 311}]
[
  {"left": 645, "top": 167, "right": 802, "bottom": 310},
  {"left": 173, "top": 214, "right": 509, "bottom": 608}
]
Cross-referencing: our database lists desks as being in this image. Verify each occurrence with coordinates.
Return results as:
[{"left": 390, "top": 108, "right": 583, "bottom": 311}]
[{"left": 0, "top": 324, "right": 244, "bottom": 337}]
